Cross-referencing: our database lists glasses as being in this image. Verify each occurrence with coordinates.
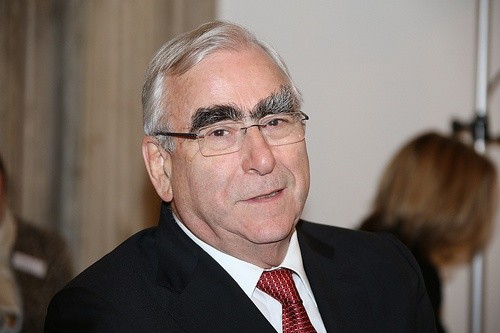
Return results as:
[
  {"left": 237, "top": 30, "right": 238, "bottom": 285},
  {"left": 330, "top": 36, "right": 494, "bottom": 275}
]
[{"left": 151, "top": 110, "right": 309, "bottom": 157}]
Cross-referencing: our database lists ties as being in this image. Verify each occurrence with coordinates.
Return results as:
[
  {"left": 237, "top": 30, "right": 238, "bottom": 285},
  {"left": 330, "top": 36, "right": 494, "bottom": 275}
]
[{"left": 256, "top": 268, "right": 317, "bottom": 333}]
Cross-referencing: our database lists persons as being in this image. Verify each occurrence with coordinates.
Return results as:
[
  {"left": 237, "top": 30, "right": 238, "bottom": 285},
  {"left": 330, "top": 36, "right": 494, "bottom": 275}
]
[
  {"left": 358, "top": 132, "right": 500, "bottom": 333},
  {"left": 0, "top": 158, "right": 70, "bottom": 333},
  {"left": 41, "top": 21, "right": 435, "bottom": 333}
]
